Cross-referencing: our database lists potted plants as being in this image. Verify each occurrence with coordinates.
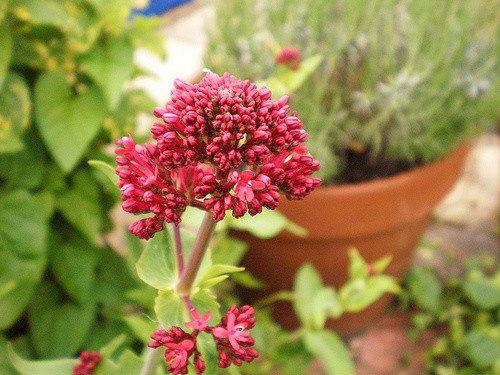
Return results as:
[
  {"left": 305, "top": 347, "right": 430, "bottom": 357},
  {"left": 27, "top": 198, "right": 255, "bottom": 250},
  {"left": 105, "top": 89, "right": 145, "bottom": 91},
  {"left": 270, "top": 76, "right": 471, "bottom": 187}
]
[{"left": 191, "top": 0, "right": 500, "bottom": 339}]
